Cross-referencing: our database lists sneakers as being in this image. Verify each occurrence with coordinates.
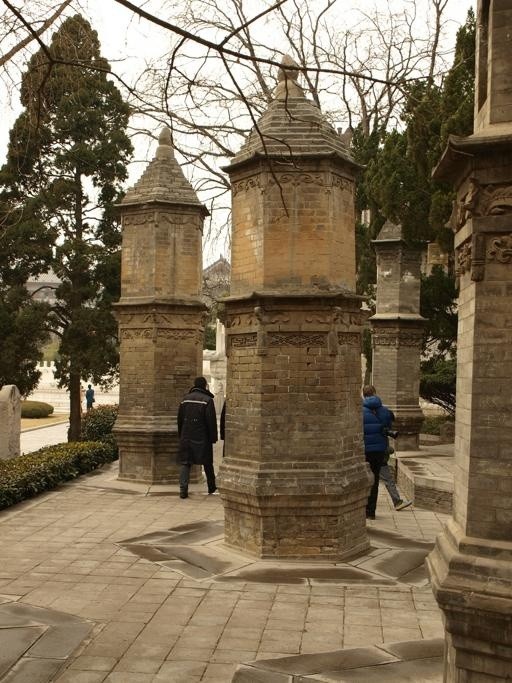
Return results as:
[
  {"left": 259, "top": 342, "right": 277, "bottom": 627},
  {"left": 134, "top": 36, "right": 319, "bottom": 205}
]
[{"left": 392, "top": 501, "right": 413, "bottom": 511}]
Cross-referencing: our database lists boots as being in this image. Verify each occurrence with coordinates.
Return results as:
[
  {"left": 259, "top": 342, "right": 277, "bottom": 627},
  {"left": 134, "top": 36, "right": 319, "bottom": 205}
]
[
  {"left": 179, "top": 491, "right": 189, "bottom": 499},
  {"left": 207, "top": 485, "right": 217, "bottom": 494}
]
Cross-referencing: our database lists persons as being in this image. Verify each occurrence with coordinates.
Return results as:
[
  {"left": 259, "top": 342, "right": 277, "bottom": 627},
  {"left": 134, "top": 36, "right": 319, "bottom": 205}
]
[
  {"left": 86, "top": 385, "right": 95, "bottom": 411},
  {"left": 177, "top": 377, "right": 217, "bottom": 498},
  {"left": 363, "top": 384, "right": 413, "bottom": 520}
]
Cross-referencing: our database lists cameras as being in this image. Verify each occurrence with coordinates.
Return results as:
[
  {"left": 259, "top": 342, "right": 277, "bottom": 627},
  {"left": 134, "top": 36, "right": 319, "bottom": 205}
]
[{"left": 381, "top": 425, "right": 400, "bottom": 439}]
